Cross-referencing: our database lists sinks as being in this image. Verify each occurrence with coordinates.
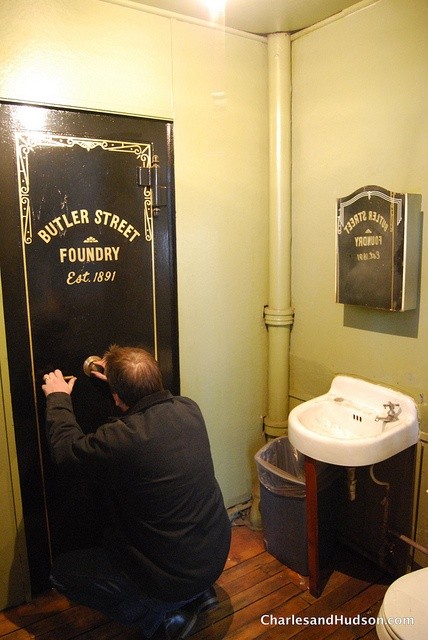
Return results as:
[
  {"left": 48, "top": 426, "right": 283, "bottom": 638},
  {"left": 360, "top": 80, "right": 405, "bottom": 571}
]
[{"left": 288, "top": 375, "right": 417, "bottom": 446}]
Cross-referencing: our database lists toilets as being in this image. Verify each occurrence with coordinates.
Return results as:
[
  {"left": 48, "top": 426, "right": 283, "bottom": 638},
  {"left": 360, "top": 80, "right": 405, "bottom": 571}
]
[{"left": 374, "top": 565, "right": 427, "bottom": 640}]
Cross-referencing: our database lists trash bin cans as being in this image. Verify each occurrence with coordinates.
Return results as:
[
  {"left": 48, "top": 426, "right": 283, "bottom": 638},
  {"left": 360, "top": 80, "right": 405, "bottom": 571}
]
[{"left": 254, "top": 436, "right": 348, "bottom": 577}]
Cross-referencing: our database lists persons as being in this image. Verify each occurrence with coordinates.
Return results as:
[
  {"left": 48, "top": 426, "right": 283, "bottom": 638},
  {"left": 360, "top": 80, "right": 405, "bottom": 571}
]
[{"left": 41, "top": 342, "right": 232, "bottom": 640}]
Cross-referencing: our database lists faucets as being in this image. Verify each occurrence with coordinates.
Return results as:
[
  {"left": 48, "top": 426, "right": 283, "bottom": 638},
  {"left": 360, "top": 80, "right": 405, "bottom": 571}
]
[{"left": 374, "top": 402, "right": 400, "bottom": 423}]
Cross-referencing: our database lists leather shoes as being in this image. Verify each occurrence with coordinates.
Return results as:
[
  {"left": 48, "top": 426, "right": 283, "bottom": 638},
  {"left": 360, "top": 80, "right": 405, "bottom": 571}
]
[
  {"left": 151, "top": 611, "right": 198, "bottom": 640},
  {"left": 182, "top": 588, "right": 219, "bottom": 619}
]
